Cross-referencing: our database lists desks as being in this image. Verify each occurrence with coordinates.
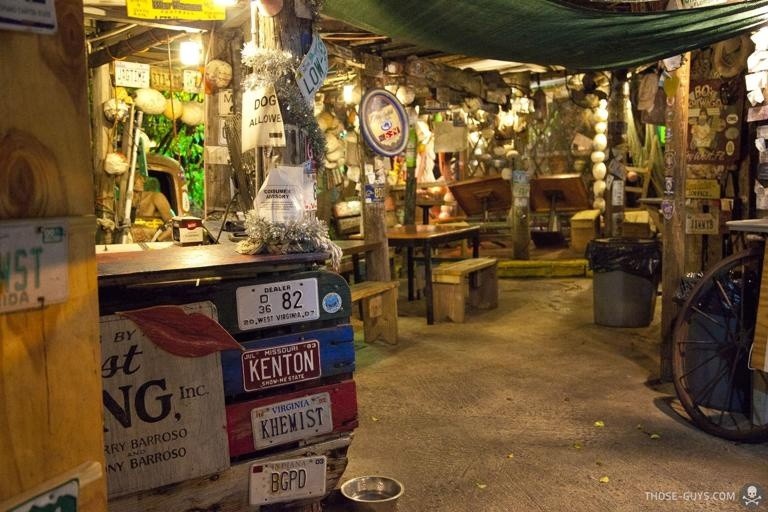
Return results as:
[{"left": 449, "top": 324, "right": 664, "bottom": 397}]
[
  {"left": 348, "top": 223, "right": 480, "bottom": 326},
  {"left": 415, "top": 173, "right": 591, "bottom": 251},
  {"left": 324, "top": 239, "right": 380, "bottom": 323}
]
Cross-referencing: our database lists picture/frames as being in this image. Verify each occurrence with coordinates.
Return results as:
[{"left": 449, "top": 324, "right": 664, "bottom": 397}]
[{"left": 358, "top": 87, "right": 410, "bottom": 157}]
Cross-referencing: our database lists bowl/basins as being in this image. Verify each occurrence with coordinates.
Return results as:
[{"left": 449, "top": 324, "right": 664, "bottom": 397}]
[{"left": 340, "top": 475, "right": 403, "bottom": 512}]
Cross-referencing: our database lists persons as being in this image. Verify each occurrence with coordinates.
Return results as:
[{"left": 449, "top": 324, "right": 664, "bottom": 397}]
[{"left": 692, "top": 108, "right": 711, "bottom": 146}]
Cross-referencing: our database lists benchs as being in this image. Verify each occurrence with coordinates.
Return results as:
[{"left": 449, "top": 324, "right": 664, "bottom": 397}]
[
  {"left": 432, "top": 257, "right": 498, "bottom": 321},
  {"left": 570, "top": 207, "right": 601, "bottom": 255},
  {"left": 624, "top": 210, "right": 649, "bottom": 241},
  {"left": 348, "top": 280, "right": 400, "bottom": 346}
]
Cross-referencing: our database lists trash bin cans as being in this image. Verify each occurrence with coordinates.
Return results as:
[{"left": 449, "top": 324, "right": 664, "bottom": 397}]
[
  {"left": 679, "top": 271, "right": 761, "bottom": 413},
  {"left": 584, "top": 237, "right": 662, "bottom": 327}
]
